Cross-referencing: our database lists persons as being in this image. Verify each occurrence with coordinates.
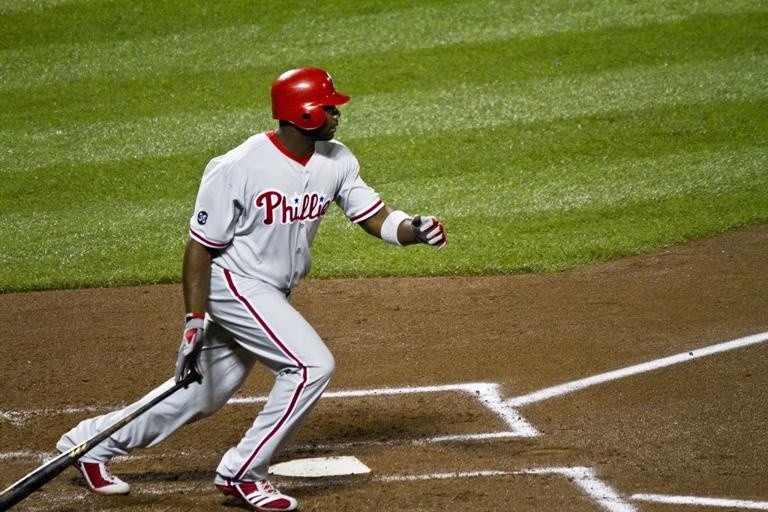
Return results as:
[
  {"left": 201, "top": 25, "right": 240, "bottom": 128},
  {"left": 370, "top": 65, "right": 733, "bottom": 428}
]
[{"left": 64, "top": 66, "right": 448, "bottom": 510}]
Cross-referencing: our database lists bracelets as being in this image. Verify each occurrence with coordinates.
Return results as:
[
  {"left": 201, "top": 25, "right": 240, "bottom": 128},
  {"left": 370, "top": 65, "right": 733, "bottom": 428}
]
[{"left": 379, "top": 210, "right": 413, "bottom": 248}]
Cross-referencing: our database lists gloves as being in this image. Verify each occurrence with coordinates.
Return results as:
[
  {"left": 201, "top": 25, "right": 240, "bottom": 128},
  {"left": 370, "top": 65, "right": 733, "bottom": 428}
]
[
  {"left": 411, "top": 214, "right": 448, "bottom": 251},
  {"left": 175, "top": 312, "right": 205, "bottom": 389}
]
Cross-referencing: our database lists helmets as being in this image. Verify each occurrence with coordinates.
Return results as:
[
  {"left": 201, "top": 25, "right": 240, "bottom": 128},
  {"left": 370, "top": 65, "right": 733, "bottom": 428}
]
[{"left": 271, "top": 67, "right": 351, "bottom": 131}]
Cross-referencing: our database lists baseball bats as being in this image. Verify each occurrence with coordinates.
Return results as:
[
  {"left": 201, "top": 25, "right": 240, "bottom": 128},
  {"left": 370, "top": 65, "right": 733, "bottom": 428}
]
[{"left": 0, "top": 370, "right": 203, "bottom": 510}]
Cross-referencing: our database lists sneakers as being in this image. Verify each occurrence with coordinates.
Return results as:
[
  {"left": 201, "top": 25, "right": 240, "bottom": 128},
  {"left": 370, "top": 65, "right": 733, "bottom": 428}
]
[
  {"left": 214, "top": 473, "right": 298, "bottom": 512},
  {"left": 56, "top": 424, "right": 131, "bottom": 495}
]
